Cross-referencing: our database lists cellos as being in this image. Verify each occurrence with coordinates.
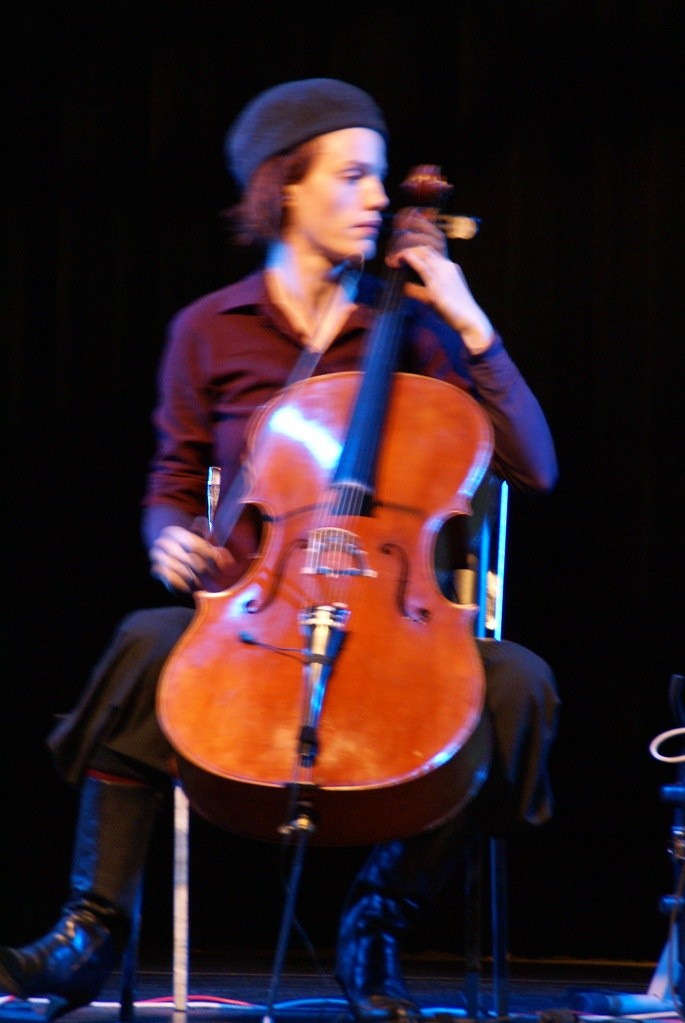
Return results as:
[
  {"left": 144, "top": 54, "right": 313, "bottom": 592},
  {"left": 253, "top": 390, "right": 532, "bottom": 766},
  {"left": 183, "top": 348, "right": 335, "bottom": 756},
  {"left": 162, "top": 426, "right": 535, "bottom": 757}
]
[{"left": 152, "top": 161, "right": 513, "bottom": 1009}]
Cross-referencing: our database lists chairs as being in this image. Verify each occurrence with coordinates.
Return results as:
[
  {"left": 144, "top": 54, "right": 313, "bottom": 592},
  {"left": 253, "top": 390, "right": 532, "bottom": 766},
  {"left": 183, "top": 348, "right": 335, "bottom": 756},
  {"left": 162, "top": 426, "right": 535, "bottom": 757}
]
[{"left": 118, "top": 473, "right": 518, "bottom": 1022}]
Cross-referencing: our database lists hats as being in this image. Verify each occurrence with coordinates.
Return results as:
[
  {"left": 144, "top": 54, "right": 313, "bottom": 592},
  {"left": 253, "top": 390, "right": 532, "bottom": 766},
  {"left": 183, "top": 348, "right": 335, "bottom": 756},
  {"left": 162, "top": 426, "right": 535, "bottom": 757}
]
[{"left": 226, "top": 78, "right": 386, "bottom": 188}]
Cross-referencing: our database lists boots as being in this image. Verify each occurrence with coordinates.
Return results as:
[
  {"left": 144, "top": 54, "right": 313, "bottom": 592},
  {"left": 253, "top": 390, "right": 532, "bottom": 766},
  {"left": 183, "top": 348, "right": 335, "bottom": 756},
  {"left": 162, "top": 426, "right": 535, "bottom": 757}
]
[
  {"left": 326, "top": 839, "right": 420, "bottom": 1020},
  {"left": 0, "top": 773, "right": 156, "bottom": 1006}
]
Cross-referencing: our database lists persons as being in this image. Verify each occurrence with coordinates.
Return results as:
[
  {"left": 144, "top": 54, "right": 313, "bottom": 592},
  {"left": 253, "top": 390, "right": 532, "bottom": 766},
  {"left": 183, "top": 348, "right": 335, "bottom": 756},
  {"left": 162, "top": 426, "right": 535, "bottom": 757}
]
[{"left": 0, "top": 80, "right": 559, "bottom": 1023}]
